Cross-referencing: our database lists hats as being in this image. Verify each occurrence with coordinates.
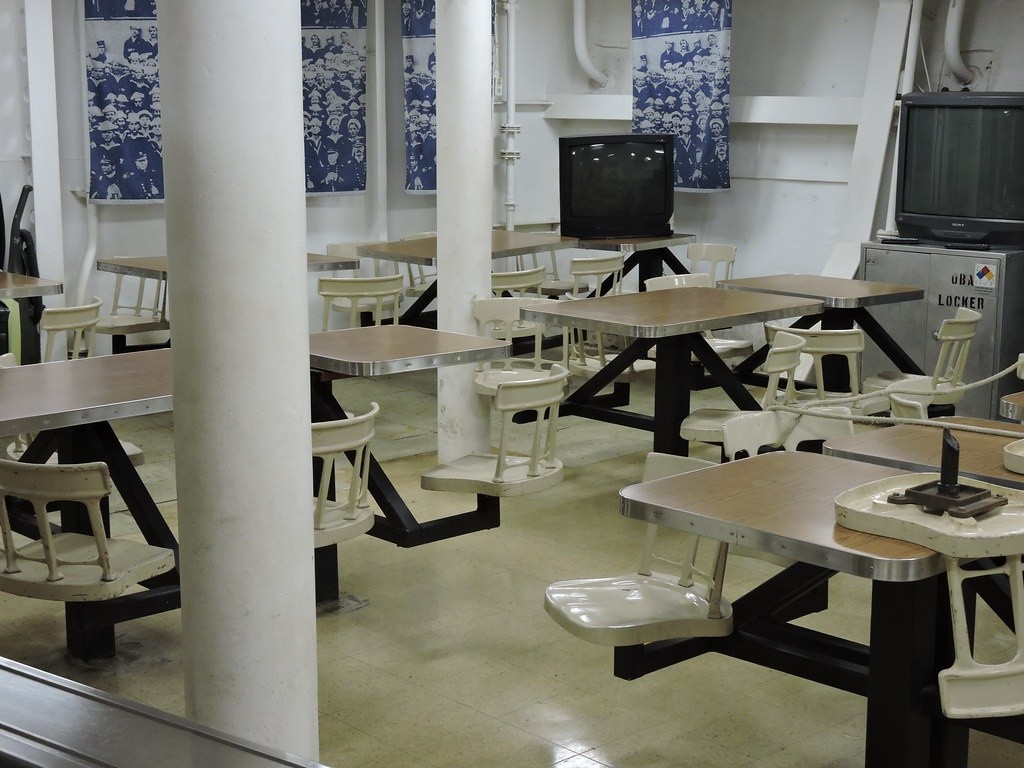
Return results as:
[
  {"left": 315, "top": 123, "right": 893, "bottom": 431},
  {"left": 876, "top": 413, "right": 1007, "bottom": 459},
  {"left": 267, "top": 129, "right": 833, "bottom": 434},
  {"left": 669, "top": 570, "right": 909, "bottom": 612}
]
[
  {"left": 84, "top": 25, "right": 161, "bottom": 166},
  {"left": 301, "top": 0, "right": 437, "bottom": 163},
  {"left": 633, "top": 0, "right": 729, "bottom": 150}
]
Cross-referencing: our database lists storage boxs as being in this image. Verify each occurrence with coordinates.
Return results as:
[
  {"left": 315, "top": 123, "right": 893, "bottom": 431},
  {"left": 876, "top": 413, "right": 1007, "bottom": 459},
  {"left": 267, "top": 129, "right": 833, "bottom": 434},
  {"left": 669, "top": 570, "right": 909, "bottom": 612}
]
[{"left": 859, "top": 237, "right": 1024, "bottom": 425}]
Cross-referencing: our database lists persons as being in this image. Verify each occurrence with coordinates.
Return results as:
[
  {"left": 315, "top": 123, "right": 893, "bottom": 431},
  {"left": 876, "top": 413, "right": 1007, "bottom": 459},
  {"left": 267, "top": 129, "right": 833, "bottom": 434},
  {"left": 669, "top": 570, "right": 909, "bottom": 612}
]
[
  {"left": 301, "top": 0, "right": 368, "bottom": 192},
  {"left": 632, "top": 0, "right": 732, "bottom": 189},
  {"left": 85, "top": 24, "right": 165, "bottom": 200},
  {"left": 401, "top": 0, "right": 437, "bottom": 190}
]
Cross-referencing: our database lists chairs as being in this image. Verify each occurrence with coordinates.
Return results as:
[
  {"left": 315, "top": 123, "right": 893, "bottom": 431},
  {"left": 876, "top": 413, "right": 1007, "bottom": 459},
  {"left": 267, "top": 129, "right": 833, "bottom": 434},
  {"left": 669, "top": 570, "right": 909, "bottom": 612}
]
[
  {"left": 319, "top": 274, "right": 404, "bottom": 328},
  {"left": 519, "top": 231, "right": 590, "bottom": 294},
  {"left": 686, "top": 241, "right": 737, "bottom": 287},
  {"left": 0, "top": 352, "right": 147, "bottom": 469},
  {"left": 307, "top": 402, "right": 380, "bottom": 545},
  {"left": 402, "top": 232, "right": 438, "bottom": 297},
  {"left": 644, "top": 272, "right": 754, "bottom": 362},
  {"left": 678, "top": 329, "right": 807, "bottom": 442},
  {"left": 566, "top": 251, "right": 629, "bottom": 300},
  {"left": 91, "top": 255, "right": 170, "bottom": 335},
  {"left": 0, "top": 457, "right": 177, "bottom": 602},
  {"left": 473, "top": 297, "right": 569, "bottom": 399},
  {"left": 491, "top": 266, "right": 548, "bottom": 338},
  {"left": 39, "top": 295, "right": 103, "bottom": 363},
  {"left": 325, "top": 241, "right": 405, "bottom": 309},
  {"left": 761, "top": 322, "right": 891, "bottom": 422},
  {"left": 720, "top": 406, "right": 854, "bottom": 463},
  {"left": 424, "top": 362, "right": 568, "bottom": 494},
  {"left": 862, "top": 306, "right": 983, "bottom": 419},
  {"left": 543, "top": 450, "right": 738, "bottom": 640},
  {"left": 838, "top": 427, "right": 1024, "bottom": 718}
]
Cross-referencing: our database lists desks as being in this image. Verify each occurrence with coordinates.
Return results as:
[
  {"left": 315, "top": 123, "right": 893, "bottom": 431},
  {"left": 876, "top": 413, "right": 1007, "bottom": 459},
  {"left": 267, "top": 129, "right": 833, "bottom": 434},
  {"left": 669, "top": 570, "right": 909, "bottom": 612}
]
[{"left": 0, "top": 229, "right": 1024, "bottom": 768}]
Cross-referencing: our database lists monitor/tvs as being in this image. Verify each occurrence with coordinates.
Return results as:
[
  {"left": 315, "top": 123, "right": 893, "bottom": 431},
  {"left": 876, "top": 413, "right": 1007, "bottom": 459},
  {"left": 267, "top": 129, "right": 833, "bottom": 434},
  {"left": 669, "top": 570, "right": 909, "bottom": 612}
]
[
  {"left": 559, "top": 134, "right": 675, "bottom": 239},
  {"left": 895, "top": 92, "right": 1024, "bottom": 249}
]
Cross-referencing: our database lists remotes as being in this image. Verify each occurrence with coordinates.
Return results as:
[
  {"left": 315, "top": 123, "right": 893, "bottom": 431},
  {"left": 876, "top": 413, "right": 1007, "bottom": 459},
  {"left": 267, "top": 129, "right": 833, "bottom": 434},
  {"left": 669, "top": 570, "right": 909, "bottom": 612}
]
[
  {"left": 944, "top": 242, "right": 989, "bottom": 250},
  {"left": 881, "top": 238, "right": 919, "bottom": 244}
]
